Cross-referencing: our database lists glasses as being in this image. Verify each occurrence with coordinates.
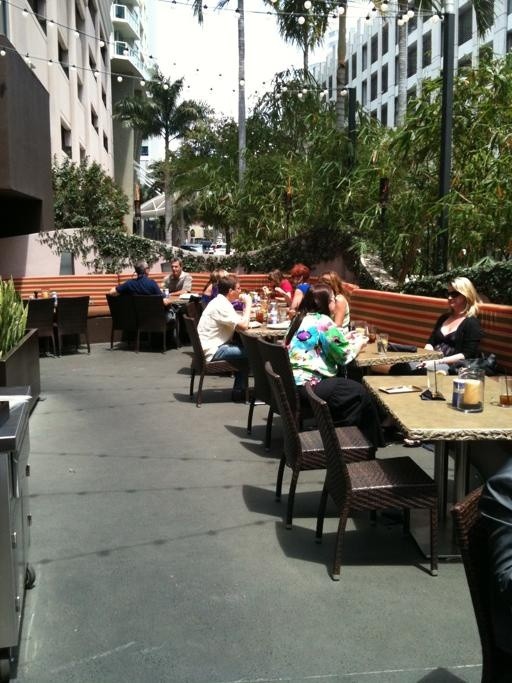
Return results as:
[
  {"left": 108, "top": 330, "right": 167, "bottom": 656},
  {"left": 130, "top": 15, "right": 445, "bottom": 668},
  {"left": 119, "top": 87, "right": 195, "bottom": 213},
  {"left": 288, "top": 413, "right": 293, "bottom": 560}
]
[
  {"left": 446, "top": 289, "right": 460, "bottom": 297},
  {"left": 268, "top": 276, "right": 273, "bottom": 281}
]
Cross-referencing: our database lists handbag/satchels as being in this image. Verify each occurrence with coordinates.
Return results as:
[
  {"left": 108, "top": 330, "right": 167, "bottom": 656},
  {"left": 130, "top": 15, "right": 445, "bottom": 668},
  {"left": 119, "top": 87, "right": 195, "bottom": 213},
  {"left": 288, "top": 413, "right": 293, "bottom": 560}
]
[{"left": 448, "top": 354, "right": 495, "bottom": 377}]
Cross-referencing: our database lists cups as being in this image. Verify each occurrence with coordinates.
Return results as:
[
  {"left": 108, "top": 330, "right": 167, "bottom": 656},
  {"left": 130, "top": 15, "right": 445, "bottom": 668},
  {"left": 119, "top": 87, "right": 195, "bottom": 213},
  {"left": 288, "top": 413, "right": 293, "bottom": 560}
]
[
  {"left": 367, "top": 325, "right": 376, "bottom": 344},
  {"left": 253, "top": 301, "right": 297, "bottom": 323},
  {"left": 426, "top": 362, "right": 512, "bottom": 412},
  {"left": 375, "top": 333, "right": 389, "bottom": 355}
]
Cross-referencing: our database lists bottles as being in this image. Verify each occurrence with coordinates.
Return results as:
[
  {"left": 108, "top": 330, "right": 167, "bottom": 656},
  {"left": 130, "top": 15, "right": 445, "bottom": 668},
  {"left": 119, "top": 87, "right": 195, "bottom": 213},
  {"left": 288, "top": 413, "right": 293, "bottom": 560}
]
[{"left": 268, "top": 301, "right": 278, "bottom": 324}]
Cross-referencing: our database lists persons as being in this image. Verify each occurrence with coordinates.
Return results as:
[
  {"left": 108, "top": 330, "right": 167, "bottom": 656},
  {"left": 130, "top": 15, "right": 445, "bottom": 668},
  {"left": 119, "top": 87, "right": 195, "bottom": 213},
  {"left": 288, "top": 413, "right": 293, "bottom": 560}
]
[
  {"left": 382, "top": 273, "right": 494, "bottom": 432},
  {"left": 106, "top": 256, "right": 352, "bottom": 403},
  {"left": 468, "top": 447, "right": 512, "bottom": 683},
  {"left": 283, "top": 283, "right": 387, "bottom": 447}
]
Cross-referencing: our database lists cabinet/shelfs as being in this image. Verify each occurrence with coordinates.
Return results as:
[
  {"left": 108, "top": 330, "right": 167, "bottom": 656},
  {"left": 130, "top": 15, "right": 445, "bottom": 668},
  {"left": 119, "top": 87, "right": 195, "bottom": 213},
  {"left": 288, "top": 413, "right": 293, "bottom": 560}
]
[{"left": 0, "top": 387, "right": 37, "bottom": 682}]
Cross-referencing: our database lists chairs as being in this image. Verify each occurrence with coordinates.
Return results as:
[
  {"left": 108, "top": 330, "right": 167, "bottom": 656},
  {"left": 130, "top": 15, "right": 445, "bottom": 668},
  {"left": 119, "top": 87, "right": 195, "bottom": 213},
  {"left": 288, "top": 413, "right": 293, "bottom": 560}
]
[{"left": 450, "top": 483, "right": 512, "bottom": 683}]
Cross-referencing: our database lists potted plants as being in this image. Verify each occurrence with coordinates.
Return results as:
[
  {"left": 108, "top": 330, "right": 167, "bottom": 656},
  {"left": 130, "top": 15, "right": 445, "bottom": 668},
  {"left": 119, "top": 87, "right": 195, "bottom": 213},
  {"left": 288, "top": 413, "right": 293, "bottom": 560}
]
[{"left": 0, "top": 274, "right": 42, "bottom": 414}]
